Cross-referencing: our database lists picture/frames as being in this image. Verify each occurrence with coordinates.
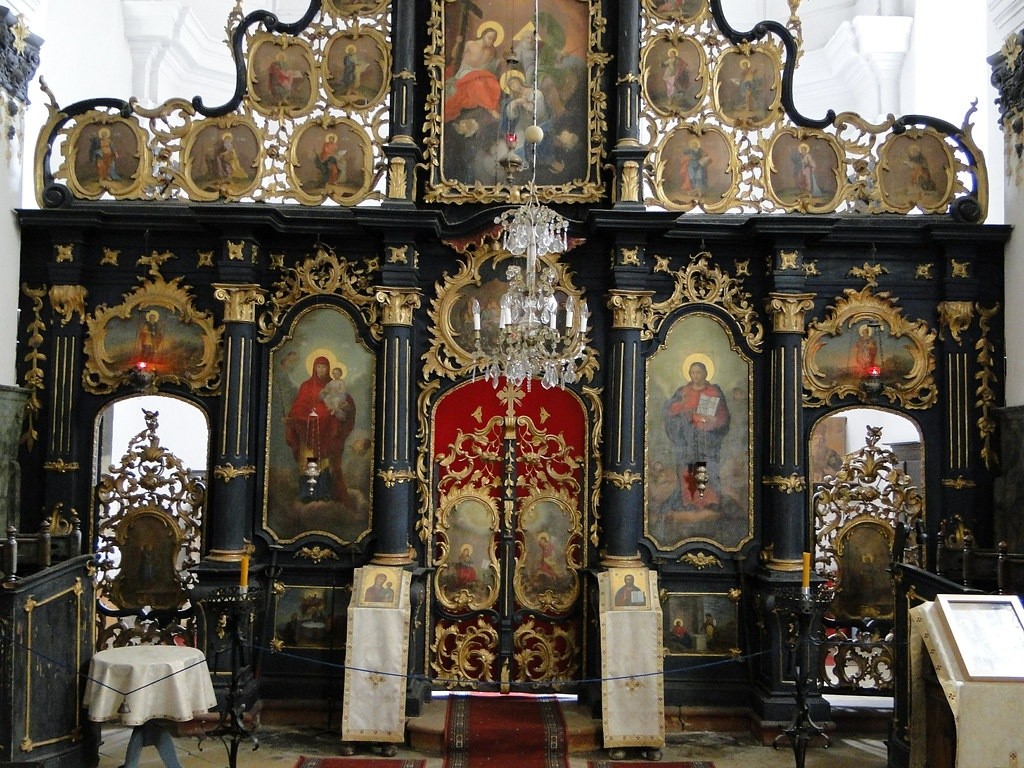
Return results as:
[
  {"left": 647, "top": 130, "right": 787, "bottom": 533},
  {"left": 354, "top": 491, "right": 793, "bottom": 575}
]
[
  {"left": 272, "top": 585, "right": 351, "bottom": 652},
  {"left": 357, "top": 565, "right": 403, "bottom": 609},
  {"left": 659, "top": 591, "right": 740, "bottom": 659},
  {"left": 939, "top": 593, "right": 1024, "bottom": 683},
  {"left": 609, "top": 566, "right": 651, "bottom": 612}
]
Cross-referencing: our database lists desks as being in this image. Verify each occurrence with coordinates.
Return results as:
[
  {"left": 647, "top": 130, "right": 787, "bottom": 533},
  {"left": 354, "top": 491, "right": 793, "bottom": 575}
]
[{"left": 83, "top": 645, "right": 218, "bottom": 768}]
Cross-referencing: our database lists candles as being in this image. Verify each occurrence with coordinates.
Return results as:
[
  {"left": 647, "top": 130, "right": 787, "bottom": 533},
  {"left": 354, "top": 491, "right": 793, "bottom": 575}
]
[
  {"left": 803, "top": 552, "right": 810, "bottom": 587},
  {"left": 240, "top": 553, "right": 249, "bottom": 586}
]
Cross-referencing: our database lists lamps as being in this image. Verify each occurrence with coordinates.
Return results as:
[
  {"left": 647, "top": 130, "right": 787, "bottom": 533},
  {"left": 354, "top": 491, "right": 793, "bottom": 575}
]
[{"left": 471, "top": 0, "right": 588, "bottom": 388}]
